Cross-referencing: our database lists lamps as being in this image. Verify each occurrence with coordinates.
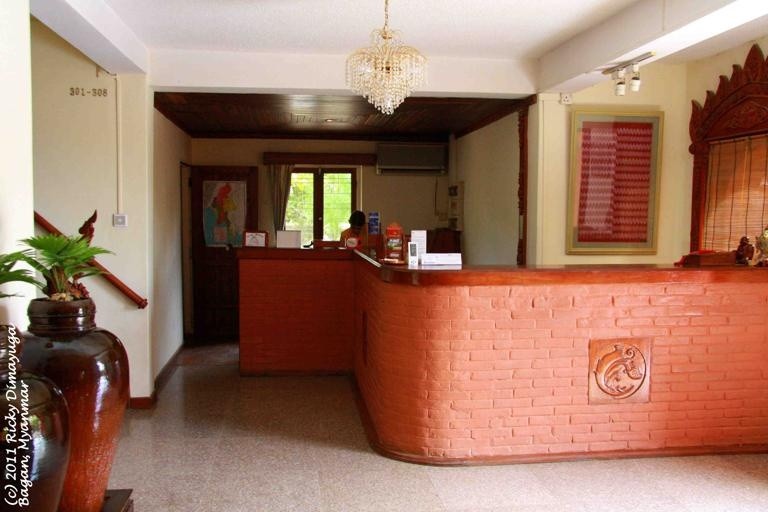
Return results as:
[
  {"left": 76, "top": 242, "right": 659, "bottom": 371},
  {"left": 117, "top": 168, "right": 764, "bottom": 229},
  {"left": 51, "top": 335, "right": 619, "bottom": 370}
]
[
  {"left": 345, "top": 0, "right": 426, "bottom": 116},
  {"left": 601, "top": 50, "right": 655, "bottom": 95}
]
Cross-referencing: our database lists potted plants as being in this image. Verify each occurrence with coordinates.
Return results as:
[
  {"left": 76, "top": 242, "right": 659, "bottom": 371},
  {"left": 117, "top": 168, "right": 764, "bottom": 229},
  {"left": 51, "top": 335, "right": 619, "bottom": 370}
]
[{"left": 0, "top": 208, "right": 130, "bottom": 512}]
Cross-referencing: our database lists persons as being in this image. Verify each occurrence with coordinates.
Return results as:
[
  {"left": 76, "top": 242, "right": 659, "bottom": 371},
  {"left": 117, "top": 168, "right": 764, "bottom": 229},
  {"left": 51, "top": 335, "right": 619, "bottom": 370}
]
[{"left": 340, "top": 210, "right": 368, "bottom": 248}]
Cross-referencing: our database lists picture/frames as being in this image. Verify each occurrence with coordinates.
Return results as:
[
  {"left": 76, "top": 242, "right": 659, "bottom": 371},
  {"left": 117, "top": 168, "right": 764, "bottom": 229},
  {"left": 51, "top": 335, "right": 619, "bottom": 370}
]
[
  {"left": 243, "top": 230, "right": 269, "bottom": 249},
  {"left": 566, "top": 110, "right": 665, "bottom": 255}
]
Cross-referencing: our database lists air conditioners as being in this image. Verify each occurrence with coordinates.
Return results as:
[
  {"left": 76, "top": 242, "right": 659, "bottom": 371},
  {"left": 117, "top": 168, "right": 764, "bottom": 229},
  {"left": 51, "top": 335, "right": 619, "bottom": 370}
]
[{"left": 376, "top": 144, "right": 446, "bottom": 176}]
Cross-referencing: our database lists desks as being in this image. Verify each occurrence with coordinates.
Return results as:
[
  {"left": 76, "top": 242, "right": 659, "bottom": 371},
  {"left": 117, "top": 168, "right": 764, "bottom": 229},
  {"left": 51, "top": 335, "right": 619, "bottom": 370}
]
[{"left": 100, "top": 488, "right": 135, "bottom": 512}]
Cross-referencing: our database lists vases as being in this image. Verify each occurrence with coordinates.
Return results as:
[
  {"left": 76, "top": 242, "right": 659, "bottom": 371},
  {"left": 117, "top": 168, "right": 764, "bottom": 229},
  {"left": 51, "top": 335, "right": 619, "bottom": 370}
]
[{"left": 0, "top": 325, "right": 72, "bottom": 511}]
